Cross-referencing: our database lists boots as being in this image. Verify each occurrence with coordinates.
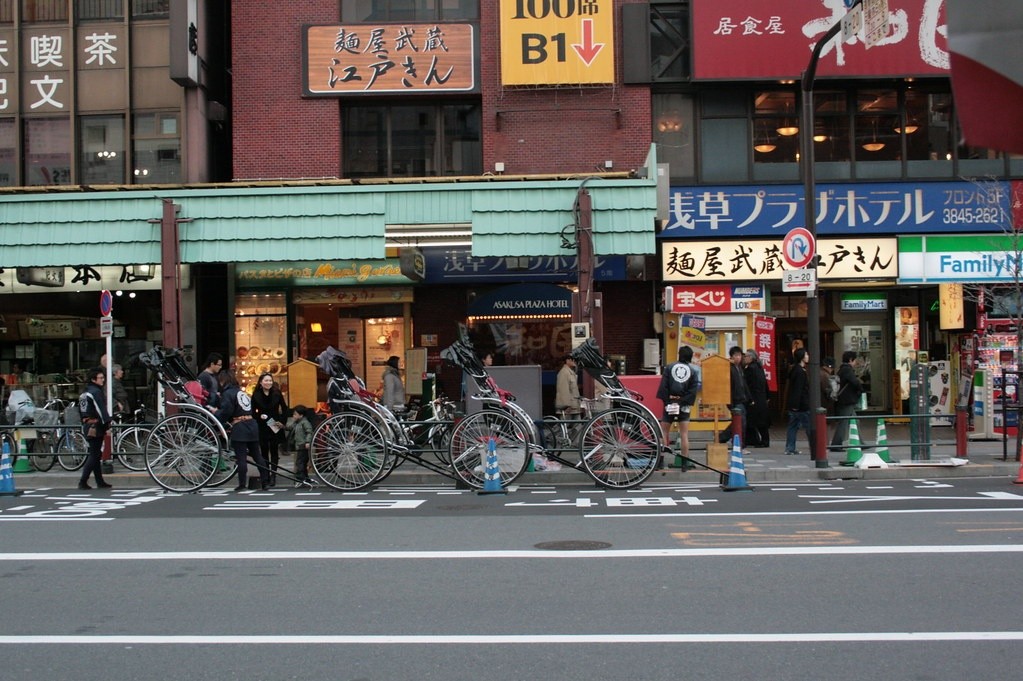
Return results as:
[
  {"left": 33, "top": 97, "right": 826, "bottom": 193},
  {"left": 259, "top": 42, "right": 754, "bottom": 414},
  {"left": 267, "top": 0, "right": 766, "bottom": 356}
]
[
  {"left": 682, "top": 459, "right": 687, "bottom": 472},
  {"left": 656, "top": 456, "right": 663, "bottom": 470}
]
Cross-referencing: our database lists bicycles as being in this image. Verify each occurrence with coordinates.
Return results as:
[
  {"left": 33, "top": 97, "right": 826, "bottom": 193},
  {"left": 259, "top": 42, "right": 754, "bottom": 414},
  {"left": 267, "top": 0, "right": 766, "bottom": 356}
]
[
  {"left": 110, "top": 399, "right": 163, "bottom": 472},
  {"left": 367, "top": 391, "right": 455, "bottom": 470},
  {"left": 32, "top": 396, "right": 89, "bottom": 472},
  {"left": 541, "top": 395, "right": 616, "bottom": 467}
]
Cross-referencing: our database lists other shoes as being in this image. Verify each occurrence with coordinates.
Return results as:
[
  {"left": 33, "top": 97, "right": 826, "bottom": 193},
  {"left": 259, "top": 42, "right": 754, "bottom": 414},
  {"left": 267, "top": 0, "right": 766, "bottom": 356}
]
[
  {"left": 785, "top": 448, "right": 802, "bottom": 455},
  {"left": 78, "top": 479, "right": 92, "bottom": 489},
  {"left": 97, "top": 481, "right": 112, "bottom": 489},
  {"left": 726, "top": 442, "right": 733, "bottom": 449},
  {"left": 830, "top": 447, "right": 845, "bottom": 452},
  {"left": 234, "top": 474, "right": 275, "bottom": 492},
  {"left": 741, "top": 449, "right": 751, "bottom": 455}
]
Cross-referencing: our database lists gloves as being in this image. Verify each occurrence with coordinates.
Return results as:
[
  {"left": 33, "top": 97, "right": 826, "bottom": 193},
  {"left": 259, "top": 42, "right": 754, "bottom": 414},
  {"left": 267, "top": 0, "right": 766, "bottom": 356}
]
[{"left": 665, "top": 403, "right": 680, "bottom": 415}]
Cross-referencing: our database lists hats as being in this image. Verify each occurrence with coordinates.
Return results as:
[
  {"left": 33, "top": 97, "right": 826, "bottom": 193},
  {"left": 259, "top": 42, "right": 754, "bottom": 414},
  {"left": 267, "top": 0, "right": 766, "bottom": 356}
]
[{"left": 823, "top": 356, "right": 837, "bottom": 368}]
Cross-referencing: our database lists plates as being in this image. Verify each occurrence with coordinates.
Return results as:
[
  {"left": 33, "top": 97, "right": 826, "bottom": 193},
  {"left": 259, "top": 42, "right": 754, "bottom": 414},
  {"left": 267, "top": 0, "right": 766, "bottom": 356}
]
[{"left": 238, "top": 345, "right": 287, "bottom": 396}]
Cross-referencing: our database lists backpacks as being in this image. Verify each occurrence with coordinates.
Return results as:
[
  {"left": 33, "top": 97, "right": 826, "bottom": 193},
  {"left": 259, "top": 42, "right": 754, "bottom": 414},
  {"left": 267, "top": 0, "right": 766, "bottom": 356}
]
[{"left": 825, "top": 375, "right": 849, "bottom": 403}]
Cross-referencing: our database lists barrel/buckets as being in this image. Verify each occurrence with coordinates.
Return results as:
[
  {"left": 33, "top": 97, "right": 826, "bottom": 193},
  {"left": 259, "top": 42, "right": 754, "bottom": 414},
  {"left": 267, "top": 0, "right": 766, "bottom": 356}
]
[{"left": 606, "top": 353, "right": 626, "bottom": 376}]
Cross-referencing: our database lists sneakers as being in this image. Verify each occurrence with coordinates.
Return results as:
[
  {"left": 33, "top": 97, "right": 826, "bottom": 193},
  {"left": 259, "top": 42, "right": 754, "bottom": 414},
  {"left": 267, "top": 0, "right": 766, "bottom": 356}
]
[{"left": 295, "top": 479, "right": 306, "bottom": 488}]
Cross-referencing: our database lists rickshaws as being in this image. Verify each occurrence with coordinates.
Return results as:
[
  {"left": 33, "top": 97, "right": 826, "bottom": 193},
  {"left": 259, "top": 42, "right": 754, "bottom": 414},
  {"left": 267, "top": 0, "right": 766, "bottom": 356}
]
[
  {"left": 568, "top": 336, "right": 731, "bottom": 490},
  {"left": 439, "top": 340, "right": 619, "bottom": 490},
  {"left": 307, "top": 345, "right": 475, "bottom": 492},
  {"left": 139, "top": 344, "right": 320, "bottom": 494}
]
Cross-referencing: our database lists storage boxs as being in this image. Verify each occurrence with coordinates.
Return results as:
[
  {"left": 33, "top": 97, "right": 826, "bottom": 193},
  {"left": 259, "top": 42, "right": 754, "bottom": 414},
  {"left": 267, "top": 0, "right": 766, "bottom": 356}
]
[{"left": 972, "top": 373, "right": 1020, "bottom": 434}]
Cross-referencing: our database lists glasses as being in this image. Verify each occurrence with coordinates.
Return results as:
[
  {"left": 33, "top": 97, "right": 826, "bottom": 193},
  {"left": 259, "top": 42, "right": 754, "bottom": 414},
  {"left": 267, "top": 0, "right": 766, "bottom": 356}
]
[
  {"left": 215, "top": 364, "right": 222, "bottom": 367},
  {"left": 261, "top": 372, "right": 271, "bottom": 376}
]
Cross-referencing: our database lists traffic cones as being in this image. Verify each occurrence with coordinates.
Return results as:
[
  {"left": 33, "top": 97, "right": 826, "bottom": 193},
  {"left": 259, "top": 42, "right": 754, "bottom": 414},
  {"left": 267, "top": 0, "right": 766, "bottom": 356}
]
[
  {"left": 1012, "top": 437, "right": 1023, "bottom": 486},
  {"left": 668, "top": 429, "right": 696, "bottom": 469},
  {"left": 363, "top": 444, "right": 379, "bottom": 469},
  {"left": 0, "top": 442, "right": 24, "bottom": 496},
  {"left": 837, "top": 419, "right": 863, "bottom": 466},
  {"left": 875, "top": 419, "right": 899, "bottom": 463},
  {"left": 859, "top": 393, "right": 868, "bottom": 412},
  {"left": 13, "top": 439, "right": 36, "bottom": 473},
  {"left": 722, "top": 434, "right": 755, "bottom": 492},
  {"left": 211, "top": 451, "right": 230, "bottom": 472},
  {"left": 477, "top": 439, "right": 508, "bottom": 494}
]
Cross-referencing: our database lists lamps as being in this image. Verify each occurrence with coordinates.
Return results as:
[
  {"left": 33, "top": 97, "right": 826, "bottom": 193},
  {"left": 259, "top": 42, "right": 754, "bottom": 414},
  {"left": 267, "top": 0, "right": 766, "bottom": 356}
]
[
  {"left": 118, "top": 265, "right": 155, "bottom": 284},
  {"left": 753, "top": 100, "right": 917, "bottom": 153}
]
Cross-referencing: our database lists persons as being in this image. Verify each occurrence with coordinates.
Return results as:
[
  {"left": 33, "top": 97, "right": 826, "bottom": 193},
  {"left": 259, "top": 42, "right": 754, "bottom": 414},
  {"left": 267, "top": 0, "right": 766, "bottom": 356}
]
[
  {"left": 902, "top": 350, "right": 916, "bottom": 371},
  {"left": 784, "top": 348, "right": 813, "bottom": 454},
  {"left": 197, "top": 352, "right": 222, "bottom": 475},
  {"left": 205, "top": 369, "right": 272, "bottom": 492},
  {"left": 76, "top": 366, "right": 113, "bottom": 490},
  {"left": 900, "top": 308, "right": 914, "bottom": 325},
  {"left": 277, "top": 404, "right": 313, "bottom": 487},
  {"left": 480, "top": 352, "right": 493, "bottom": 366},
  {"left": 656, "top": 345, "right": 699, "bottom": 472},
  {"left": 97, "top": 353, "right": 133, "bottom": 464},
  {"left": 718, "top": 345, "right": 772, "bottom": 455},
  {"left": 379, "top": 355, "right": 406, "bottom": 416},
  {"left": 250, "top": 373, "right": 288, "bottom": 484},
  {"left": 785, "top": 339, "right": 803, "bottom": 367},
  {"left": 544, "top": 353, "right": 581, "bottom": 448},
  {"left": 820, "top": 351, "right": 872, "bottom": 453},
  {"left": 896, "top": 325, "right": 912, "bottom": 342}
]
